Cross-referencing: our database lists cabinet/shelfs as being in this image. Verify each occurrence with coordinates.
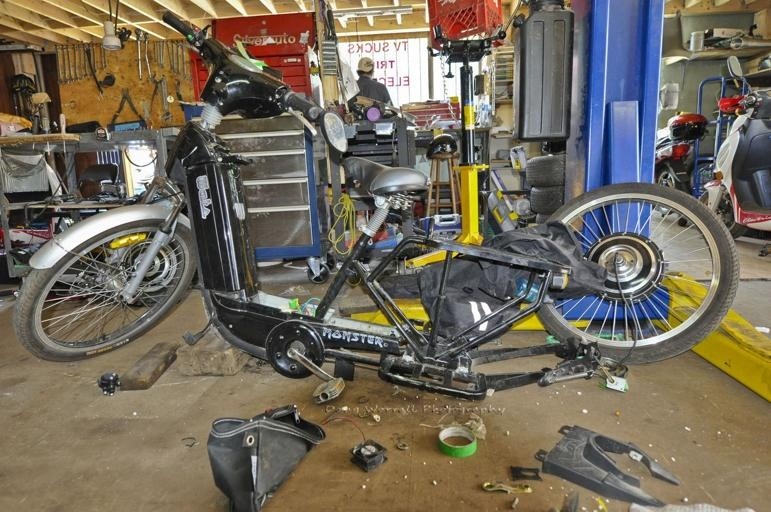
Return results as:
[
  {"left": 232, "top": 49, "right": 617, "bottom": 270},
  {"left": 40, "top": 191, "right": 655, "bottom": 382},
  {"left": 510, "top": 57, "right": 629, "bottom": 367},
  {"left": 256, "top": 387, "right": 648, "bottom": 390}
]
[{"left": 188, "top": 108, "right": 331, "bottom": 286}]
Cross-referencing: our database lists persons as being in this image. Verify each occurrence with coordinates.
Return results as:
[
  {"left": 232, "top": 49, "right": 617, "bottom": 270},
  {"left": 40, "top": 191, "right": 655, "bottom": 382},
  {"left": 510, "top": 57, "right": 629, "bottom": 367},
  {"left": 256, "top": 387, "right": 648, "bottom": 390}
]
[{"left": 348, "top": 57, "right": 393, "bottom": 118}]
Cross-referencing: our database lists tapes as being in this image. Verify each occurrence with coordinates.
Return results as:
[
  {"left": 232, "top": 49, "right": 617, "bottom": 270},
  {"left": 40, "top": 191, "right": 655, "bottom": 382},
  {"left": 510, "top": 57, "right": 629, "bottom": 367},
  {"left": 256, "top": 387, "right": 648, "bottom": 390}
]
[{"left": 438, "top": 426, "right": 477, "bottom": 458}]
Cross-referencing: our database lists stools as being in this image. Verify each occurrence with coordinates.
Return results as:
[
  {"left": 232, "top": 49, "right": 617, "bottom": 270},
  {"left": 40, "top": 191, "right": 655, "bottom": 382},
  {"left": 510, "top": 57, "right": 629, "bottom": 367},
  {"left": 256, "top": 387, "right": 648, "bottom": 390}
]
[{"left": 426, "top": 152, "right": 461, "bottom": 217}]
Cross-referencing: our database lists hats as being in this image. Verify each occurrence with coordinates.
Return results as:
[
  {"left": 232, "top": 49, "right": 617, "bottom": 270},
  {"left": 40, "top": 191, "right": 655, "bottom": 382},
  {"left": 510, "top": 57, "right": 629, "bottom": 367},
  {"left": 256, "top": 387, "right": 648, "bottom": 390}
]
[{"left": 358, "top": 58, "right": 374, "bottom": 72}]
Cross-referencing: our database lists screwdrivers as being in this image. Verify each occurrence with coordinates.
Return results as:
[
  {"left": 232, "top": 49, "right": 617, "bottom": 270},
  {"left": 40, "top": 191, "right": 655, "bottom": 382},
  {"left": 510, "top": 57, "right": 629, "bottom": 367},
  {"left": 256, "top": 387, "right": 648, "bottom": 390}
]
[{"left": 32, "top": 113, "right": 66, "bottom": 156}]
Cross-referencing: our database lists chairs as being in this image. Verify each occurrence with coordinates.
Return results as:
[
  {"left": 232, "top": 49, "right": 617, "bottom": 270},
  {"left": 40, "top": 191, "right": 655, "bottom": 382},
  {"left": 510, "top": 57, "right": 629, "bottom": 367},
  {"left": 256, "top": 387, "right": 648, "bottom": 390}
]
[{"left": 43, "top": 162, "right": 121, "bottom": 234}]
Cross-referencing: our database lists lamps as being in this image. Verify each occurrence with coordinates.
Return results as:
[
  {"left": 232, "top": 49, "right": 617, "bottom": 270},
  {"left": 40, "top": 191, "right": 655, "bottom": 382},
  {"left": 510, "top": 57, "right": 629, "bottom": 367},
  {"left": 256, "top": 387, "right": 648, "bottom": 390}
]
[{"left": 101, "top": 0, "right": 123, "bottom": 52}]
